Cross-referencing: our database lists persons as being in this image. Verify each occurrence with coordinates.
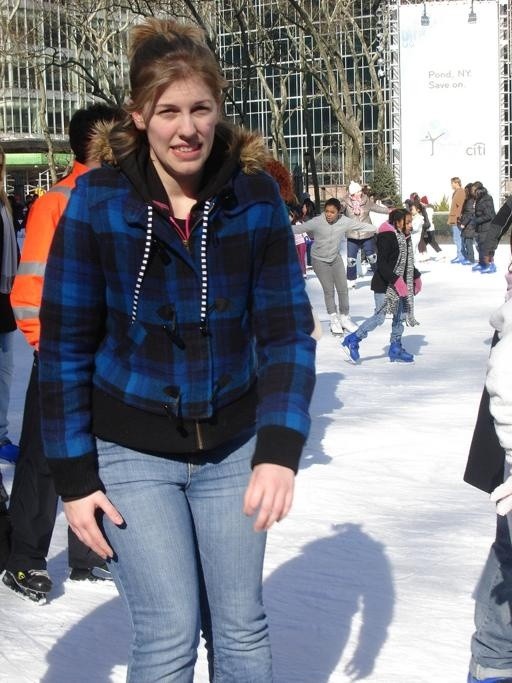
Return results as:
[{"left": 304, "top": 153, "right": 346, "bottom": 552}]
[
  {"left": 42, "top": 18, "right": 317, "bottom": 681},
  {"left": 463, "top": 258, "right": 512, "bottom": 683},
  {"left": 2, "top": 104, "right": 132, "bottom": 602},
  {"left": 290, "top": 177, "right": 497, "bottom": 362}
]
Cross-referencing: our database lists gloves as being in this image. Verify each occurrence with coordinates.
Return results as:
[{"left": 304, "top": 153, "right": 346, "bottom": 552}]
[
  {"left": 393, "top": 276, "right": 408, "bottom": 298},
  {"left": 413, "top": 276, "right": 422, "bottom": 295}
]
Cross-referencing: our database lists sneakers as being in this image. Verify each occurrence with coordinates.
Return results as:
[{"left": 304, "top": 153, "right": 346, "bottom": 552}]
[
  {"left": 360, "top": 262, "right": 373, "bottom": 276},
  {"left": 7, "top": 563, "right": 53, "bottom": 595},
  {"left": 302, "top": 264, "right": 313, "bottom": 279},
  {"left": 472, "top": 263, "right": 496, "bottom": 273},
  {"left": 69, "top": 562, "right": 114, "bottom": 581},
  {"left": 347, "top": 278, "right": 358, "bottom": 289},
  {"left": 451, "top": 256, "right": 474, "bottom": 265}
]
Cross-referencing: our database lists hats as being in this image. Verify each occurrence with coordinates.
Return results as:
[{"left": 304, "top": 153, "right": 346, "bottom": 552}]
[
  {"left": 420, "top": 196, "right": 428, "bottom": 205},
  {"left": 348, "top": 180, "right": 363, "bottom": 196}
]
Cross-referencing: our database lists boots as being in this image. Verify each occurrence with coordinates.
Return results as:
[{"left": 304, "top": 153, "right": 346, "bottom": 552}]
[
  {"left": 435, "top": 251, "right": 446, "bottom": 261},
  {"left": 388, "top": 340, "right": 414, "bottom": 362},
  {"left": 416, "top": 251, "right": 430, "bottom": 262},
  {"left": 343, "top": 331, "right": 362, "bottom": 361},
  {"left": 330, "top": 313, "right": 343, "bottom": 334},
  {"left": 339, "top": 314, "right": 360, "bottom": 333}
]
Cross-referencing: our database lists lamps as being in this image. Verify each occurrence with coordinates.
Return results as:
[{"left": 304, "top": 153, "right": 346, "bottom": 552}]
[
  {"left": 421, "top": 4, "right": 429, "bottom": 26},
  {"left": 468, "top": 0, "right": 476, "bottom": 22}
]
